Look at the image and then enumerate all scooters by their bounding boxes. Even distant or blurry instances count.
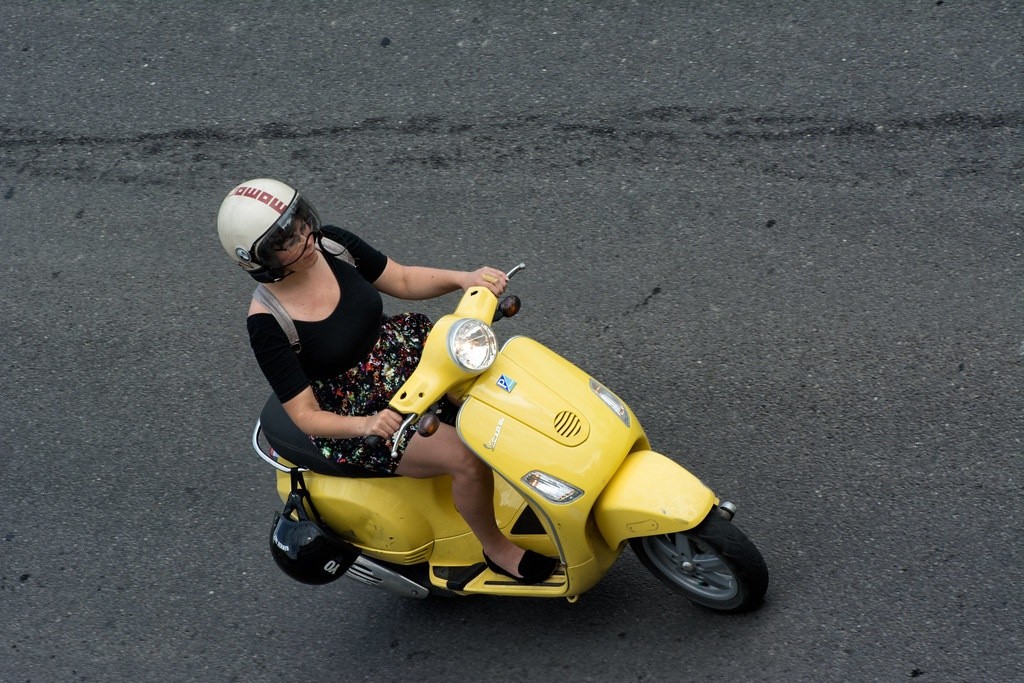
[251,261,771,620]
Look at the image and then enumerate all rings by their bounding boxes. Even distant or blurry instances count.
[482,274,499,284]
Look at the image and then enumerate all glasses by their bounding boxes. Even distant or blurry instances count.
[273,216,313,253]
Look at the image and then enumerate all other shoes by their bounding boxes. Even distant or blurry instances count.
[481,542,560,583]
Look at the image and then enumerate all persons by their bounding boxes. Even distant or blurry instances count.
[215,178,561,585]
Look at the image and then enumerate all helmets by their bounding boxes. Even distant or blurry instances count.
[270,510,362,587]
[217,177,321,285]
